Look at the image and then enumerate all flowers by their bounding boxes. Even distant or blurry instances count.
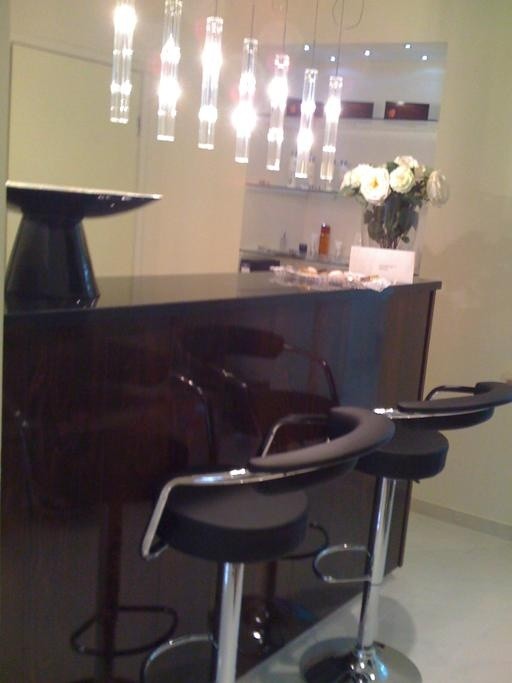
[338,155,449,249]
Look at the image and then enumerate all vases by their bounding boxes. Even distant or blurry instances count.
[350,249,417,284]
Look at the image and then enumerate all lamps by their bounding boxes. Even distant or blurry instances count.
[156,0,183,142]
[296,1,318,177]
[267,1,291,169]
[235,0,260,162]
[198,1,222,149]
[110,0,136,125]
[318,1,344,179]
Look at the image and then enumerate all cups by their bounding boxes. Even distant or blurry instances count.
[298,243,308,256]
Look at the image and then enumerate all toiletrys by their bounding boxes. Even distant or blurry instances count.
[309,231,319,258]
[299,241,308,257]
[278,227,289,256]
[332,238,343,263]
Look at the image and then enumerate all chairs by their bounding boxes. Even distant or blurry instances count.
[300,382,511,683]
[140,405,395,683]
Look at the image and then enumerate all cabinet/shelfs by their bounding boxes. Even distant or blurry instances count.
[240,111,439,271]
